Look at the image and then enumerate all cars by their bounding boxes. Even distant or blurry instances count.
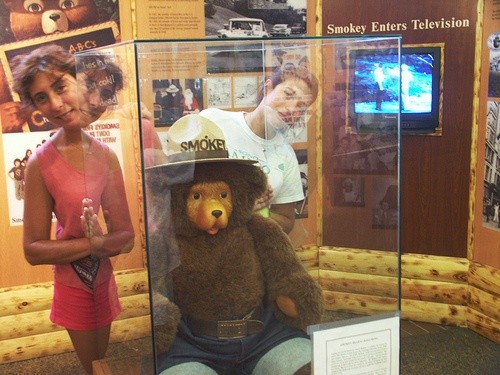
[291,23,305,34]
[270,24,291,37]
[216,17,268,38]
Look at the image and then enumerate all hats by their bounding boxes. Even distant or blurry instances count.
[144,114,258,169]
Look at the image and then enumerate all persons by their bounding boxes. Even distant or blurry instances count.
[401,64,413,110]
[342,183,359,201]
[375,63,384,110]
[14,44,90,371]
[375,201,397,224]
[74,53,136,362]
[158,84,199,121]
[8,140,46,200]
[333,117,397,170]
[485,202,499,222]
[120,65,318,233]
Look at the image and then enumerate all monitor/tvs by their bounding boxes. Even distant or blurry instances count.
[348,47,442,133]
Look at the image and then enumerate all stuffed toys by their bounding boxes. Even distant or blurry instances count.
[108,159,324,375]
